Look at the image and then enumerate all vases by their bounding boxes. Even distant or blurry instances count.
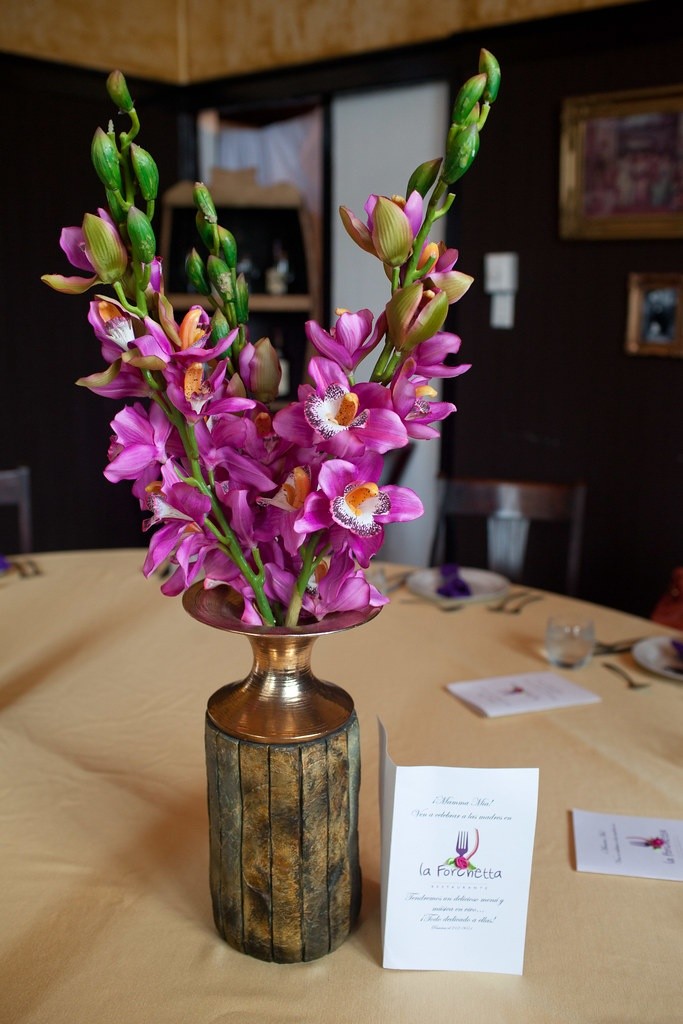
[180,577,385,966]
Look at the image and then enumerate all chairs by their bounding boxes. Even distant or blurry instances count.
[0,466,31,553]
[436,476,587,573]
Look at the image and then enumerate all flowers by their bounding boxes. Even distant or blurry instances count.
[40,47,499,626]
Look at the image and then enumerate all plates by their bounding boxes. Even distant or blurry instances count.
[407,565,512,606]
[632,635,682,680]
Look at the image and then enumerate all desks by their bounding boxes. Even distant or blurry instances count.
[0,551,683,1024]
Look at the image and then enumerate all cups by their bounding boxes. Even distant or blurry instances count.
[546,617,595,670]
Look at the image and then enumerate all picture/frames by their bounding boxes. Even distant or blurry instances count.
[625,272,683,356]
[558,85,683,239]
